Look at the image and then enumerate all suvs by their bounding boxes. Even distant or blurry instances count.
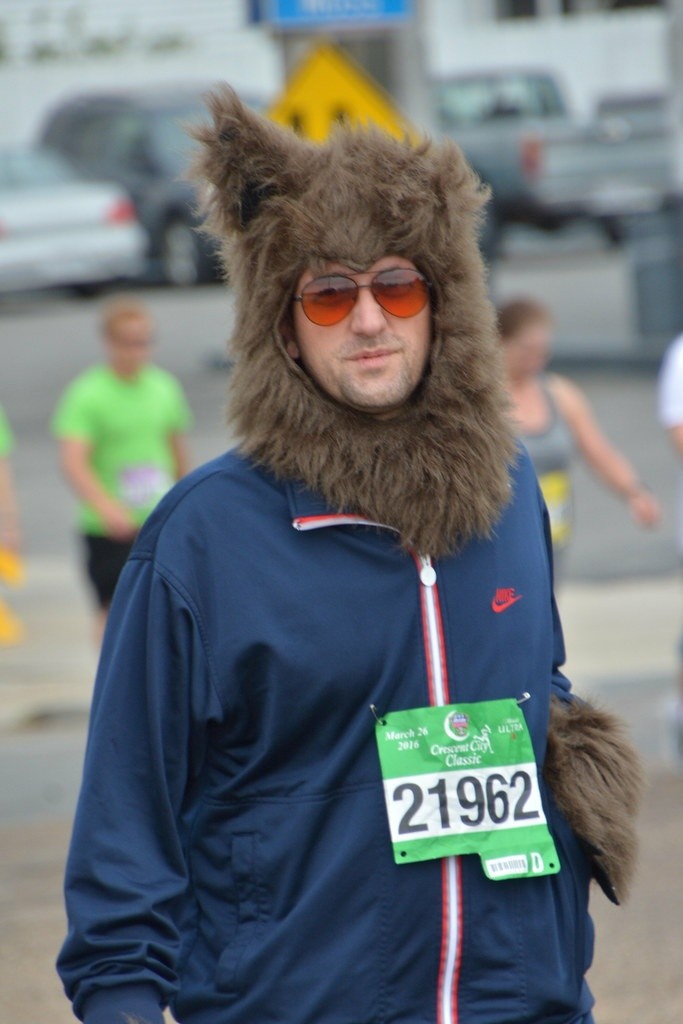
[440,70,683,251]
[2,47,277,299]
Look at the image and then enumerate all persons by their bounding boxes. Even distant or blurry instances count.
[0,408,23,551]
[55,77,660,1024]
[661,333,682,458]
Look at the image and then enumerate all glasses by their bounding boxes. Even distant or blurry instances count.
[292,268,435,327]
